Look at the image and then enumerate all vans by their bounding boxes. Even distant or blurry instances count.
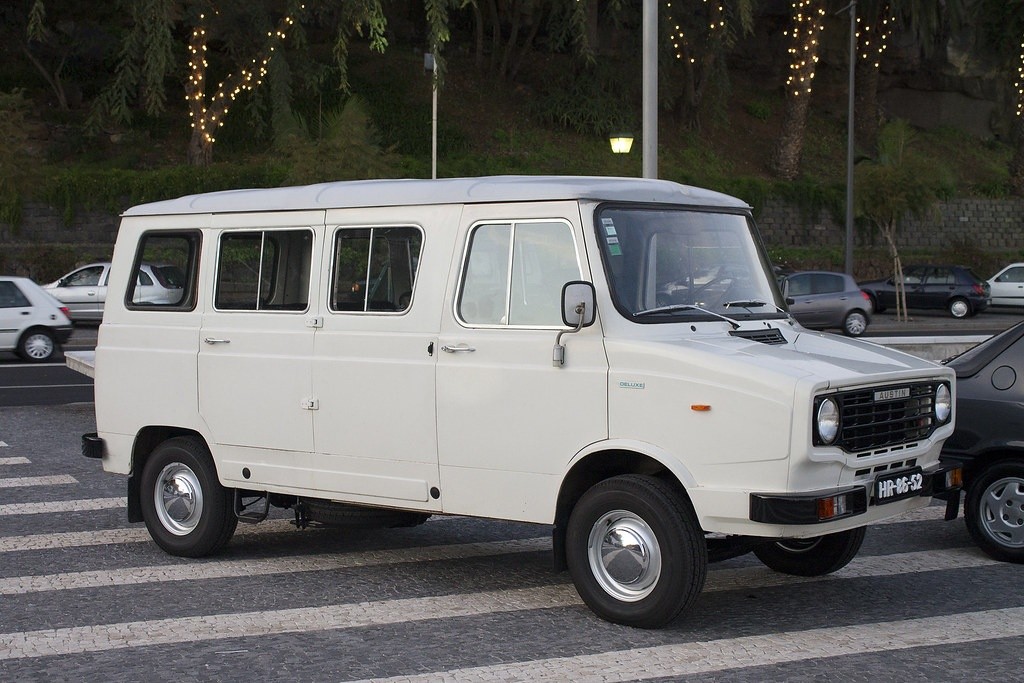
[80,173,959,625]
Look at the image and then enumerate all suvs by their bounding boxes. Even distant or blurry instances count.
[854,264,993,320]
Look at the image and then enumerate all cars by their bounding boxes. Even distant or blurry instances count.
[984,262,1024,308]
[34,261,191,322]
[778,270,874,336]
[0,275,77,361]
[928,316,1024,563]
